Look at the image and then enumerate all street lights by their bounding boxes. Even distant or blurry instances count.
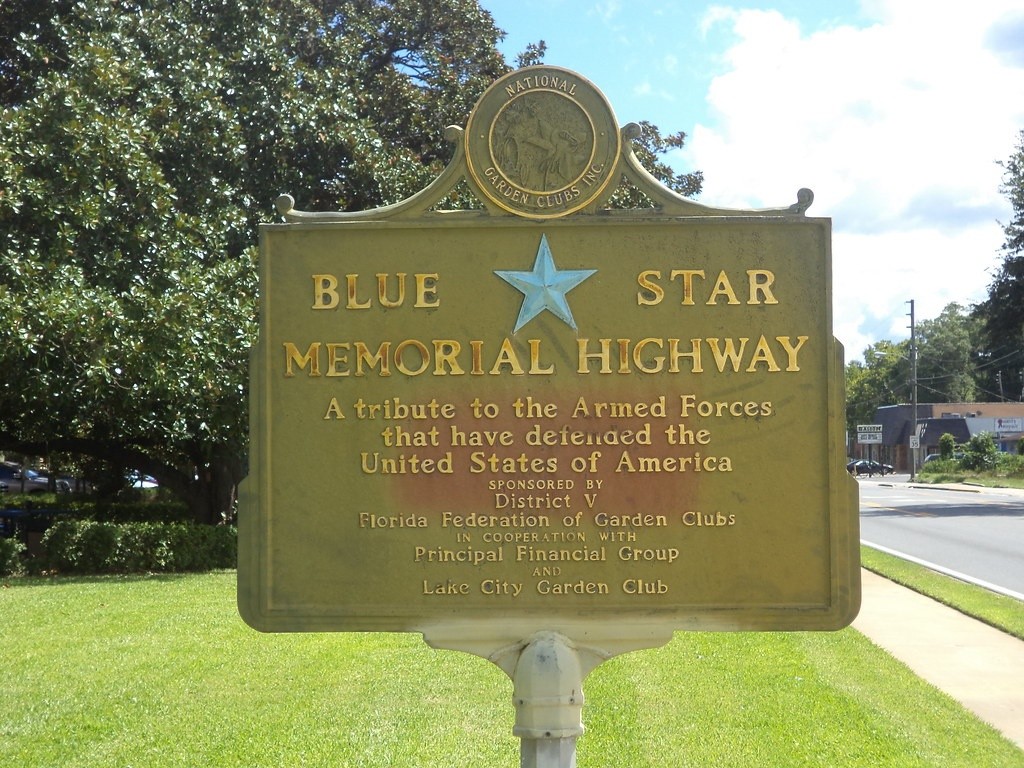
[873,351,919,483]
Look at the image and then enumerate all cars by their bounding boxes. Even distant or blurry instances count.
[854,460,893,475]
[0,461,71,497]
[846,460,860,474]
[925,454,942,464]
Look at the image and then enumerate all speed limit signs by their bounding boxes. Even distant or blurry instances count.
[909,435,919,448]
